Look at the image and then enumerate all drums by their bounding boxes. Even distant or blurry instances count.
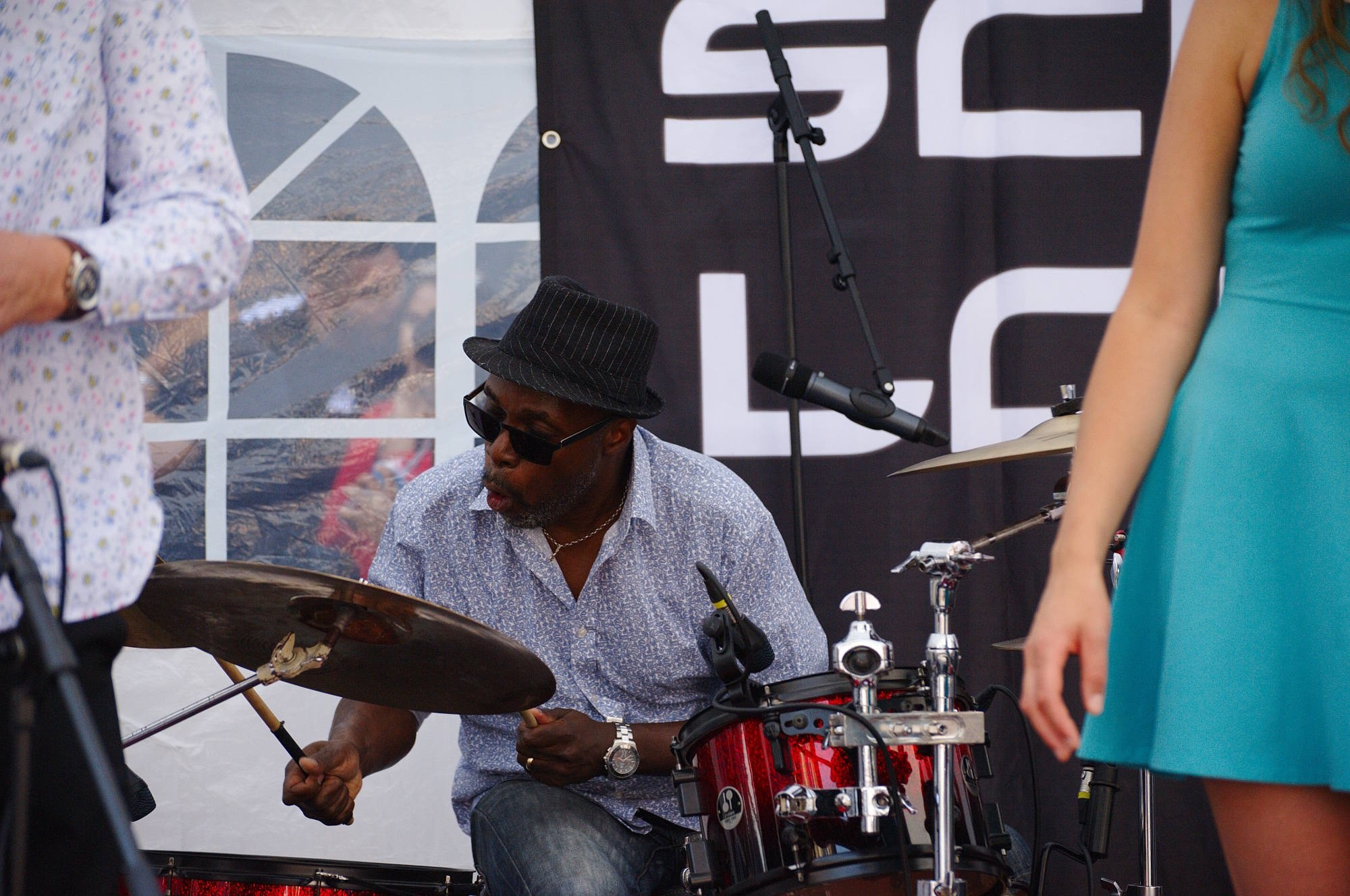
[677,665,1015,896]
[153,854,485,895]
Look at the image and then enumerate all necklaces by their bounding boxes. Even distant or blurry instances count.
[543,480,627,566]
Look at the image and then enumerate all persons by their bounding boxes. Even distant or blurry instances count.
[280,276,831,896]
[321,280,434,579]
[1019,0,1350,896]
[0,0,253,896]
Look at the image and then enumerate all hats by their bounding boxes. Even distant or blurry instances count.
[462,274,665,419]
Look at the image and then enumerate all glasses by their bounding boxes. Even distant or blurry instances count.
[463,377,617,466]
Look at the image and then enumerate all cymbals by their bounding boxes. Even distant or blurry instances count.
[884,412,1081,482]
[138,557,560,718]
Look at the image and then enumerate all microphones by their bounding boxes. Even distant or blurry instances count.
[970,684,996,712]
[0,438,50,475]
[1078,759,1096,828]
[695,561,775,673]
[752,351,950,446]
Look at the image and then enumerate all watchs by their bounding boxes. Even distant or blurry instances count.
[57,235,103,322]
[605,721,639,779]
